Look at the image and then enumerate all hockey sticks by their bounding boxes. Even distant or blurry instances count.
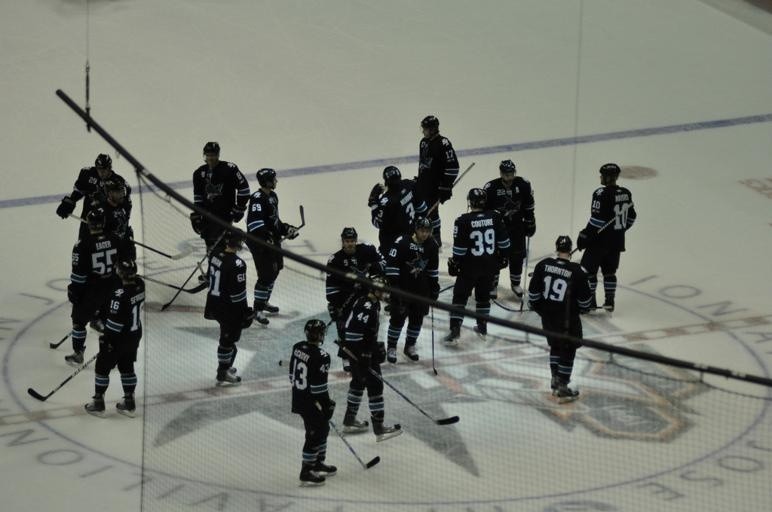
[313,400,380,471]
[431,303,440,376]
[139,274,210,294]
[49,330,72,349]
[341,346,460,426]
[278,291,355,366]
[27,356,98,401]
[273,205,305,243]
[69,213,193,261]
[519,236,531,312]
[160,218,236,311]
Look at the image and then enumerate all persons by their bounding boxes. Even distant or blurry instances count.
[204,229,255,387]
[483,159,536,299]
[412,116,460,247]
[288,320,337,486]
[190,142,250,283]
[326,226,388,361]
[529,236,595,402]
[342,274,404,441]
[65,209,128,368]
[580,164,636,311]
[90,184,137,336]
[442,188,511,345]
[85,257,144,418]
[248,168,298,328]
[56,154,132,240]
[384,214,440,367]
[367,167,429,256]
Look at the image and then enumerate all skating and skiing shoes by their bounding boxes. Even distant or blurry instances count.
[65,349,86,368]
[603,298,615,318]
[386,342,398,366]
[217,363,243,386]
[342,357,354,377]
[299,466,328,486]
[84,397,107,419]
[374,417,403,442]
[114,397,137,418]
[511,284,526,298]
[473,325,488,340]
[549,376,579,404]
[342,414,370,435]
[490,285,498,300]
[402,343,420,363]
[255,307,269,328]
[318,463,336,477]
[263,303,281,316]
[443,325,461,346]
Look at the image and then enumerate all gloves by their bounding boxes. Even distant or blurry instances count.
[328,302,341,320]
[374,341,386,364]
[240,306,254,330]
[57,196,77,219]
[523,218,536,235]
[91,313,106,333]
[430,281,441,300]
[281,224,299,238]
[190,211,207,236]
[318,398,335,420]
[577,228,598,251]
[230,207,247,222]
[68,282,87,304]
[448,258,460,275]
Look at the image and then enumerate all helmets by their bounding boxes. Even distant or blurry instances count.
[556,235,572,250]
[303,319,326,341]
[87,211,106,232]
[203,143,220,158]
[115,257,138,277]
[383,165,402,183]
[422,116,441,128]
[499,159,517,172]
[340,227,358,240]
[257,168,276,185]
[414,216,434,231]
[600,163,622,176]
[470,188,488,204]
[104,176,126,193]
[95,155,114,167]
